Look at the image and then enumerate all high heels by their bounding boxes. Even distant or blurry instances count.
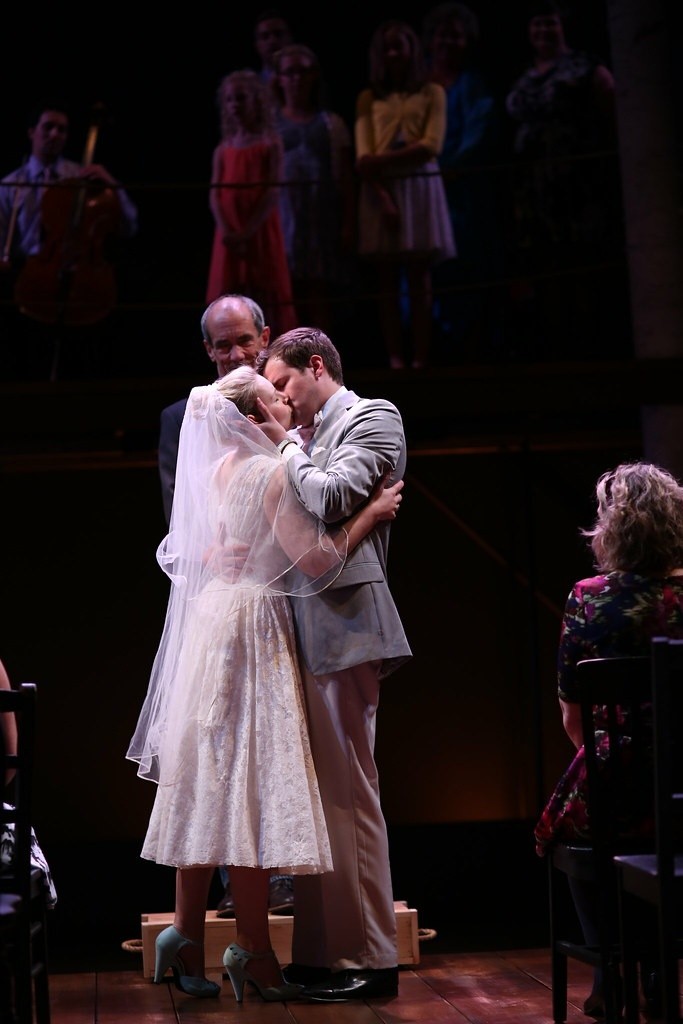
[153,926,221,998]
[223,942,305,1003]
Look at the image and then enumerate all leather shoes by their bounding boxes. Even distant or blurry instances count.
[303,969,399,1002]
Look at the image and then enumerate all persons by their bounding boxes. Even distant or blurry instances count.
[535,465,683,1024]
[1,102,139,383]
[0,658,56,1024]
[126,295,406,1004]
[210,1,614,377]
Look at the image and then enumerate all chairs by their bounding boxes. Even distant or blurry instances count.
[0,682,52,1024]
[547,637,683,1024]
